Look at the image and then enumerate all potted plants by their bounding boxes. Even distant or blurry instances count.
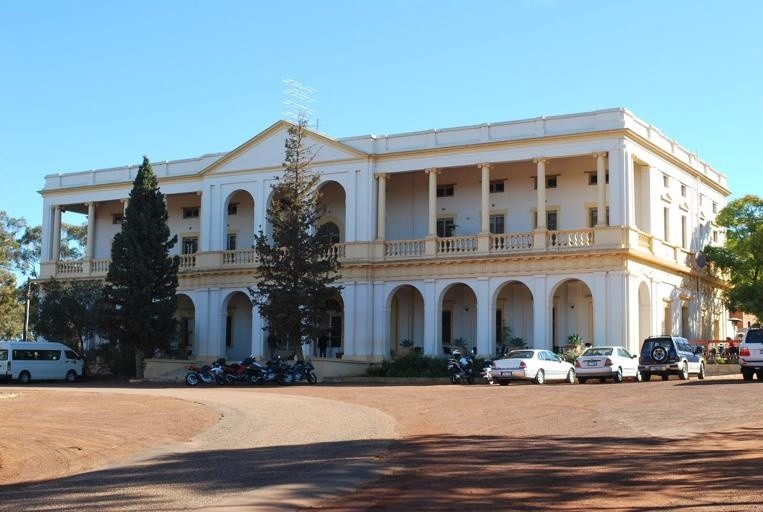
[453,337,469,356]
[564,333,584,353]
[506,337,526,352]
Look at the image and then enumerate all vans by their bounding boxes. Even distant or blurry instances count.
[0,341,86,384]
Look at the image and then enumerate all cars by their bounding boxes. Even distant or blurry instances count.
[479,335,642,386]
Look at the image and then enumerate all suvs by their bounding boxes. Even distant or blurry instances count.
[638,334,706,380]
[738,327,763,382]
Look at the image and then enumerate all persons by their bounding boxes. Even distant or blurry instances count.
[317,334,329,357]
[266,334,282,360]
[722,337,736,356]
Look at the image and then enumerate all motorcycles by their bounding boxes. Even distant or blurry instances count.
[447,350,479,384]
[185,352,317,386]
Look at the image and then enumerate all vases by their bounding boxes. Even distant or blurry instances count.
[399,340,413,356]
